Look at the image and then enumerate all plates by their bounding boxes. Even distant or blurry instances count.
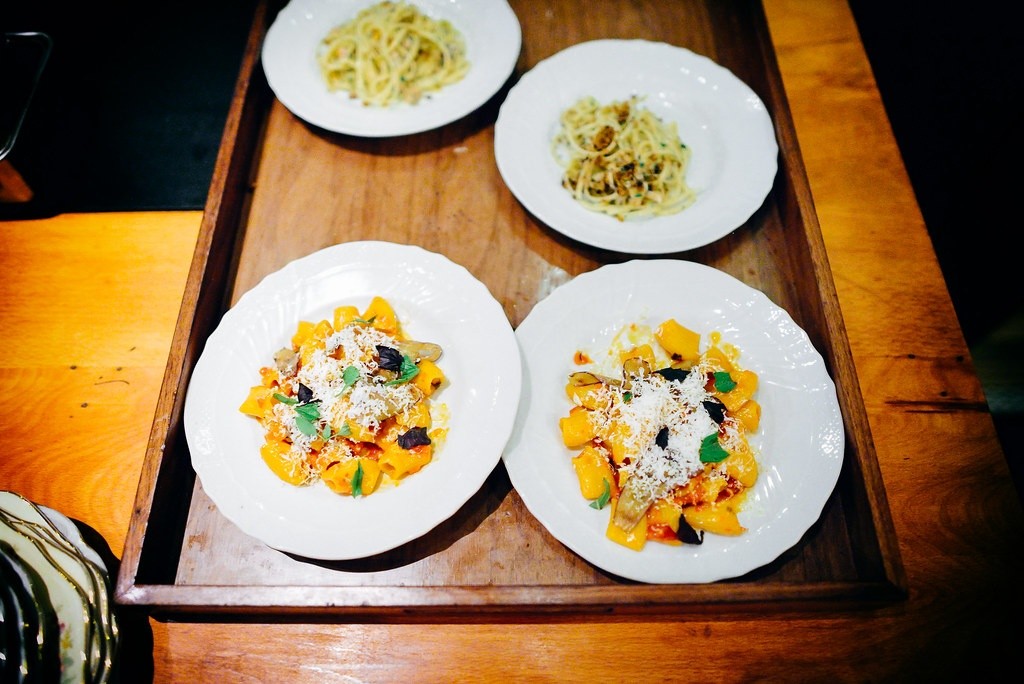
[493,39,778,254]
[500,258,845,583]
[182,241,524,562]
[0,488,119,684]
[260,0,522,138]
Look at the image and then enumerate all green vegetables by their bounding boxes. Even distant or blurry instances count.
[275,313,421,500]
[588,371,737,510]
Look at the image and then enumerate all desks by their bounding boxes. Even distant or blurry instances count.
[0,0,1024,684]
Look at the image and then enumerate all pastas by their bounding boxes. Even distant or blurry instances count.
[318,0,470,107]
[553,95,696,222]
